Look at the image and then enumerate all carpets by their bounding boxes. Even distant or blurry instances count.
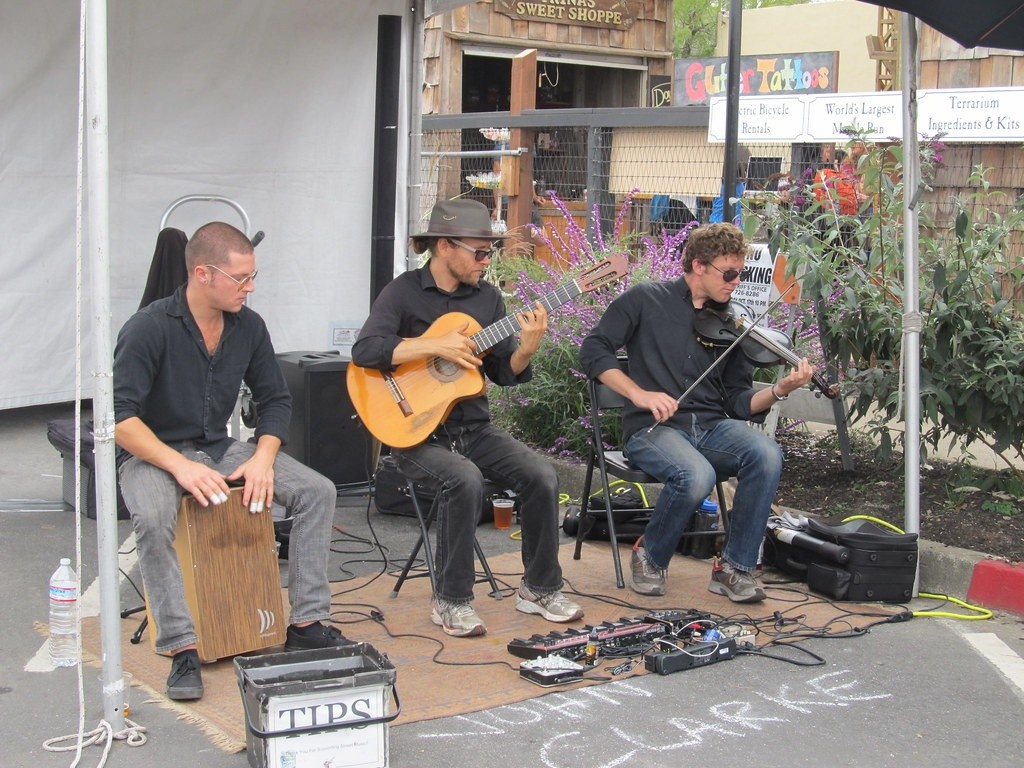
[28,531,920,757]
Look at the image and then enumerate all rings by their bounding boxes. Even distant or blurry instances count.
[652,408,657,411]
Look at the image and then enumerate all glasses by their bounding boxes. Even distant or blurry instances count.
[704,258,751,282]
[202,263,259,292]
[446,236,495,261]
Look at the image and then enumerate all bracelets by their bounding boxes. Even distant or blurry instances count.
[772,384,790,401]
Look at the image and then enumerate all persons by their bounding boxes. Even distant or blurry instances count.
[113,221,358,699]
[710,163,745,229]
[351,199,585,637]
[492,142,545,229]
[580,222,817,601]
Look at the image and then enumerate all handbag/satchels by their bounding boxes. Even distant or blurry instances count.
[801,515,917,603]
[47,418,94,476]
[585,479,697,549]
[373,451,522,526]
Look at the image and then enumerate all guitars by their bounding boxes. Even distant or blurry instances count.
[345,251,631,451]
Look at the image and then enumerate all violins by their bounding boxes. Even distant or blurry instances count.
[693,299,843,401]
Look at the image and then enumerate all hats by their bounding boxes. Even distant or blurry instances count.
[409,198,511,238]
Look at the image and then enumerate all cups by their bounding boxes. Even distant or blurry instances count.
[492,499,515,530]
[97,672,133,716]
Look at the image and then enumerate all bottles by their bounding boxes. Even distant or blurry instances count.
[690,498,720,559]
[756,541,762,570]
[48,558,78,667]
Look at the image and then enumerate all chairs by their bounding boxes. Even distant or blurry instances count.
[574,351,732,591]
[391,454,505,601]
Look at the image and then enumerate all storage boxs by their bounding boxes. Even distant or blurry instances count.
[225,640,398,768]
[140,485,289,662]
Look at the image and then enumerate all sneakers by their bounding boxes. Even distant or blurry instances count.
[285,622,360,651]
[166,645,204,700]
[515,576,585,622]
[430,591,487,637]
[630,531,669,596]
[708,556,769,604]
